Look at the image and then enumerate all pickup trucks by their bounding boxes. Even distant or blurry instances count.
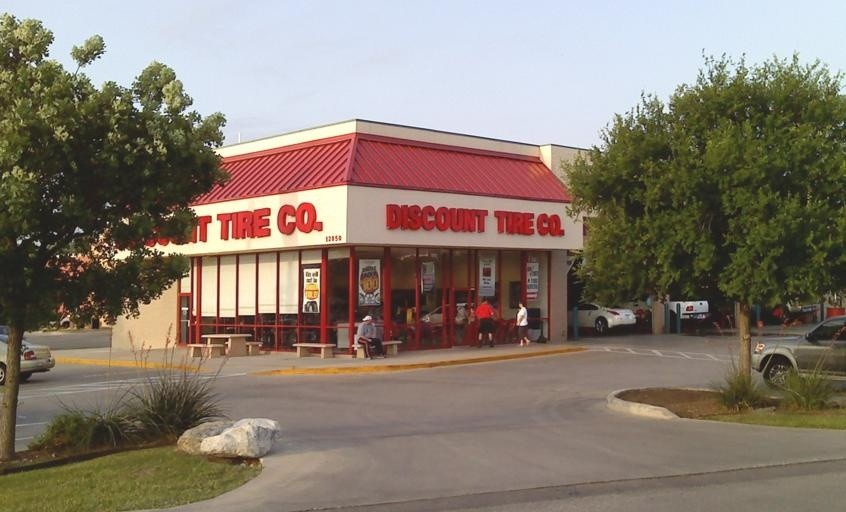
[647,293,709,320]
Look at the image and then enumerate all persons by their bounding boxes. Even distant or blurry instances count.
[514,300,531,347]
[455,307,468,336]
[356,315,384,359]
[475,297,495,349]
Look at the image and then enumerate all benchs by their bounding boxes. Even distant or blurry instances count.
[352,340,402,358]
[291,342,337,358]
[188,341,263,358]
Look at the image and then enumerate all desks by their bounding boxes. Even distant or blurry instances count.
[201,333,252,357]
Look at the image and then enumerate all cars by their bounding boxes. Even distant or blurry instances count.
[0,325,56,385]
[60,315,80,328]
[751,315,846,390]
[420,303,475,328]
[568,301,645,336]
[267,318,316,349]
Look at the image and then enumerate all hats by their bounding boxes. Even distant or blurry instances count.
[362,315,373,321]
